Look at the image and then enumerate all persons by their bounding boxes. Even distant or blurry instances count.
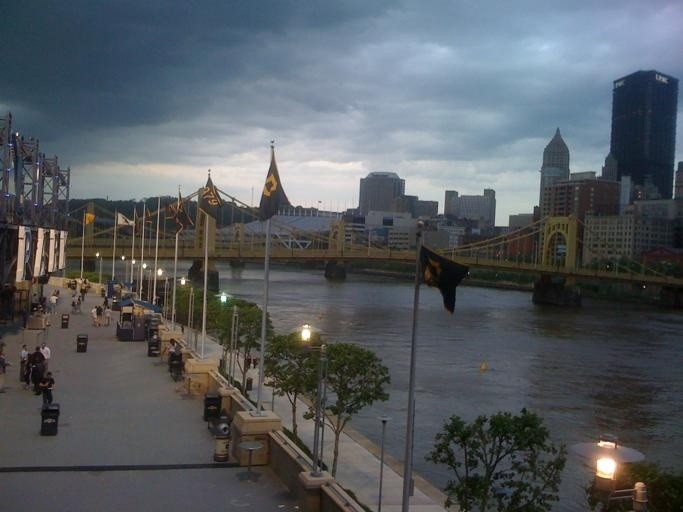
[18,344,27,381]
[28,346,45,392]
[166,338,177,376]
[38,371,55,404]
[29,278,111,327]
[38,341,51,371]
[0,343,12,394]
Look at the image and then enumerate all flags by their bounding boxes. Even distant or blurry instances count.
[134,211,142,235]
[257,149,292,221]
[175,192,193,234]
[197,177,223,221]
[117,211,134,227]
[417,244,470,317]
[85,213,98,225]
[165,202,177,219]
[144,207,151,225]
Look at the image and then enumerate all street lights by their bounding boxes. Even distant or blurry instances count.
[214,290,239,391]
[297,319,330,478]
[92,249,196,352]
[569,430,651,512]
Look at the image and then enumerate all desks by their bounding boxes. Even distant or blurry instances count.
[237,441,264,482]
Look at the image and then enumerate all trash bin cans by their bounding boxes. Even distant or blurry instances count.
[214,422,230,462]
[204,394,220,422]
[77,334,88,352]
[61,313,69,329]
[41,403,60,436]
